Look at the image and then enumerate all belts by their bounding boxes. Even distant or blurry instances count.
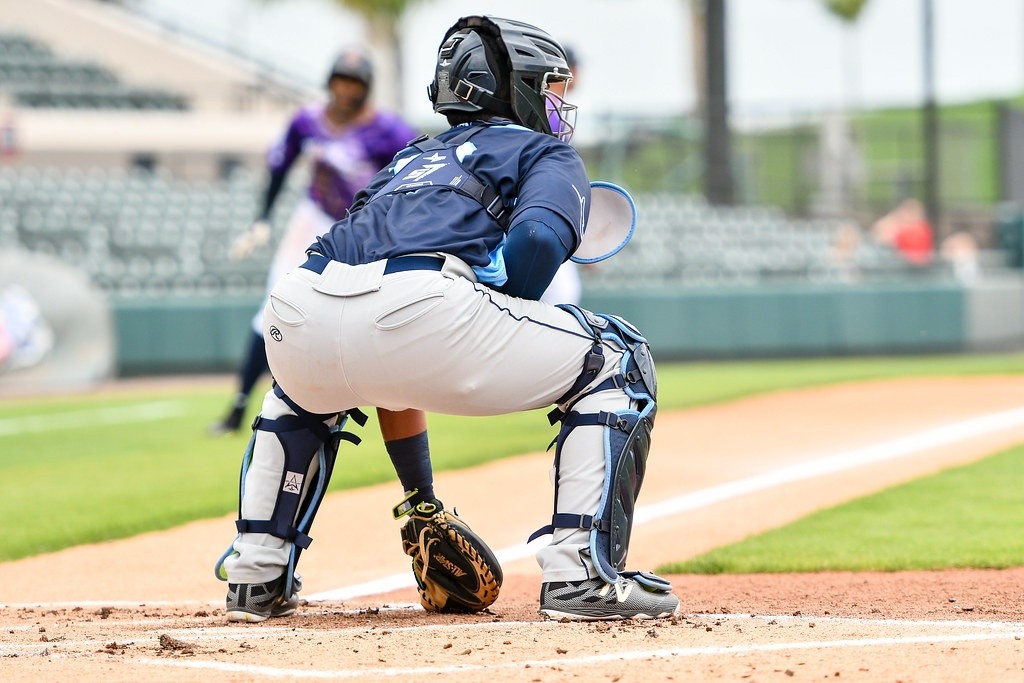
[298,254,445,275]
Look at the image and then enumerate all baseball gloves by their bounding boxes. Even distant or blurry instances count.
[394,498,504,613]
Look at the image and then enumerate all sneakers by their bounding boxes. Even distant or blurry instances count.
[536,576,680,620]
[225,577,298,622]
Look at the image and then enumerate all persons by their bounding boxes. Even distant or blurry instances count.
[214,49,421,437]
[217,12,681,619]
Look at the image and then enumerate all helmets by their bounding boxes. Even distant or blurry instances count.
[332,51,374,86]
[426,15,578,143]
